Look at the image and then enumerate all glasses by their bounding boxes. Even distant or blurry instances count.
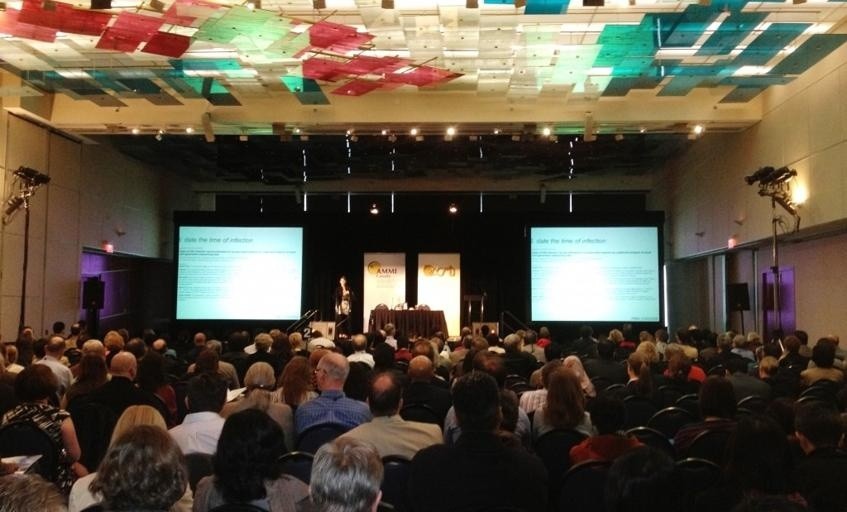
[314,369,327,374]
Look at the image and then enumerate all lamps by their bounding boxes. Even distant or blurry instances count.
[347,125,555,213]
[132,125,194,141]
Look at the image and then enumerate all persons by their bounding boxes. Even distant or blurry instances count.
[335,276,352,339]
[0,322,847,512]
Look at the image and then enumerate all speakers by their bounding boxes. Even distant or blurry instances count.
[727,282,751,311]
[82,276,105,309]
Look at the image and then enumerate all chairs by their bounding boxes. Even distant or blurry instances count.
[0,343,847,512]
